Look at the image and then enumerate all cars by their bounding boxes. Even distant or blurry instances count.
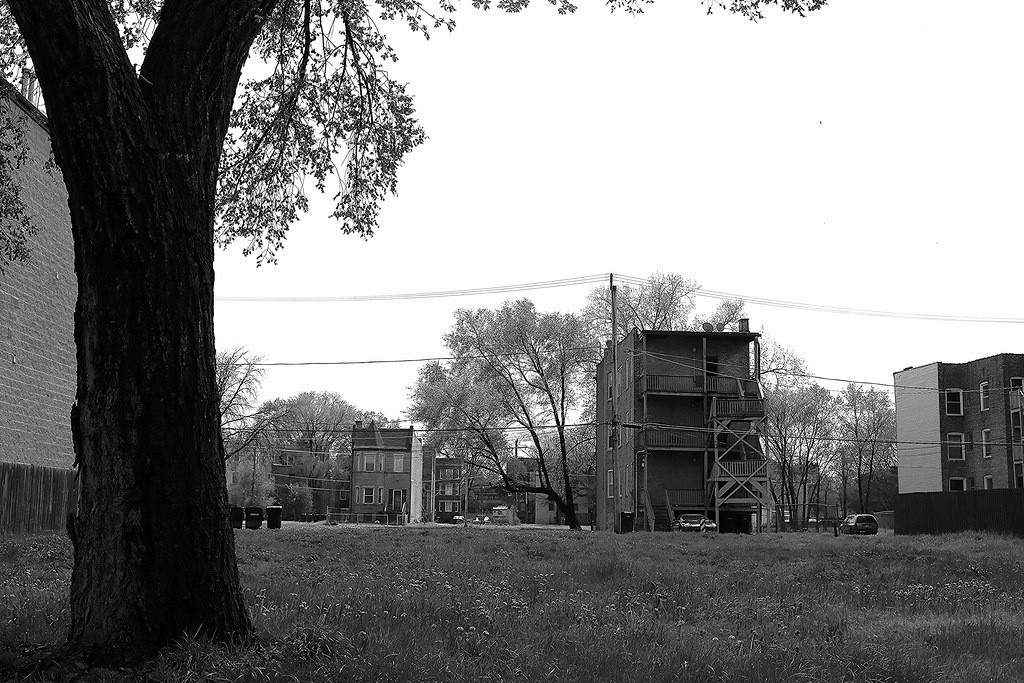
[673,515,717,533]
[453,515,464,525]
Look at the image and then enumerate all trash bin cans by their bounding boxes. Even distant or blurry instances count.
[245,505,262,530]
[621,511,634,533]
[267,506,282,529]
[230,504,244,530]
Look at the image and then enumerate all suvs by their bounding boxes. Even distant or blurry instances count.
[842,514,879,536]
